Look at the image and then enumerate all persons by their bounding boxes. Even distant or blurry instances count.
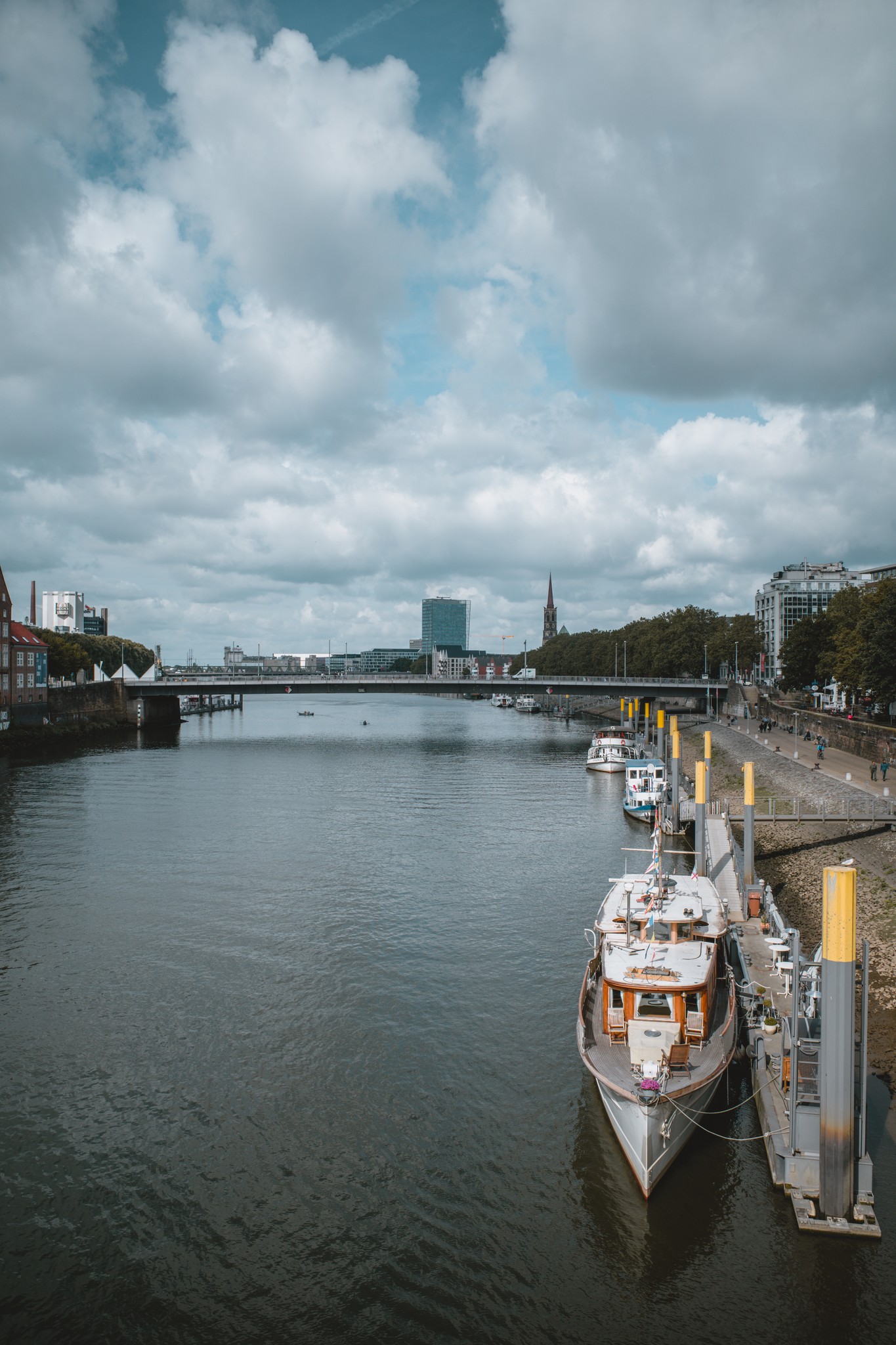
[304,711,310,713]
[869,761,878,782]
[764,717,768,723]
[865,703,874,711]
[321,672,324,680]
[726,714,737,726]
[771,720,776,726]
[712,709,715,717]
[880,759,890,781]
[725,677,728,685]
[754,701,758,711]
[743,678,746,686]
[802,729,812,741]
[756,679,758,687]
[333,672,344,680]
[758,721,772,733]
[788,725,794,733]
[817,743,825,755]
[364,721,367,724]
[813,734,826,747]
[847,713,854,721]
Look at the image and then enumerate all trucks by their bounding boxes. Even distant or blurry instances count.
[512,668,536,680]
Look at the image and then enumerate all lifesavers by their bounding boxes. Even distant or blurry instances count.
[560,708,562,711]
[197,695,198,696]
[621,739,625,745]
[598,739,601,746]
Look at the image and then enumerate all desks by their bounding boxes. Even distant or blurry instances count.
[765,938,785,970]
[775,962,793,998]
[768,945,790,978]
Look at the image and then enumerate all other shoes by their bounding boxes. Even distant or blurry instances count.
[874,780,876,781]
[883,779,885,781]
[871,779,873,781]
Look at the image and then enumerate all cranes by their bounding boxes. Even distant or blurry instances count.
[467,635,514,654]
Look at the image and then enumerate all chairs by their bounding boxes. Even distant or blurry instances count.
[684,1011,705,1052]
[661,1044,691,1080]
[607,1008,628,1047]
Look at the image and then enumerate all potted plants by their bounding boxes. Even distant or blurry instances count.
[725,908,731,926]
[637,783,642,792]
[758,908,769,935]
[656,782,660,792]
[755,986,778,1035]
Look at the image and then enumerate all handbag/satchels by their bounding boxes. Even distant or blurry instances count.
[758,728,760,732]
[766,726,769,729]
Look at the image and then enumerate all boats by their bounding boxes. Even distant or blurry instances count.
[586,726,743,1201]
[179,694,226,710]
[462,693,577,718]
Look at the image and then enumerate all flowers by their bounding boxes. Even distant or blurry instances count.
[640,1079,659,1091]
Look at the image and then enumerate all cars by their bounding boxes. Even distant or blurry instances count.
[247,671,326,672]
[372,671,411,673]
[175,671,215,673]
[738,679,752,687]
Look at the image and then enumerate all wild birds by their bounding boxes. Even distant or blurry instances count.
[840,858,855,868]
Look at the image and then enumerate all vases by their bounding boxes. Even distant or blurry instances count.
[642,1089,657,1096]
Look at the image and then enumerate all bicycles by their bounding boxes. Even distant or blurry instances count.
[817,750,824,760]
[332,676,340,681]
[622,680,728,684]
[711,715,721,722]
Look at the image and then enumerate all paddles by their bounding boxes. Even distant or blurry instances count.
[360,722,370,724]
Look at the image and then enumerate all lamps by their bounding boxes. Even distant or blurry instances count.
[681,991,687,1003]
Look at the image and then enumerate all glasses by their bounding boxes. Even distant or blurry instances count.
[873,762,875,763]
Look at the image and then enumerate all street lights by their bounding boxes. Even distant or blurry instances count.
[328,639,347,679]
[426,638,437,680]
[710,694,713,718]
[792,711,800,752]
[233,641,236,681]
[735,642,739,681]
[746,700,750,729]
[704,645,708,674]
[122,642,125,679]
[615,644,618,682]
[624,641,627,679]
[257,643,260,680]
